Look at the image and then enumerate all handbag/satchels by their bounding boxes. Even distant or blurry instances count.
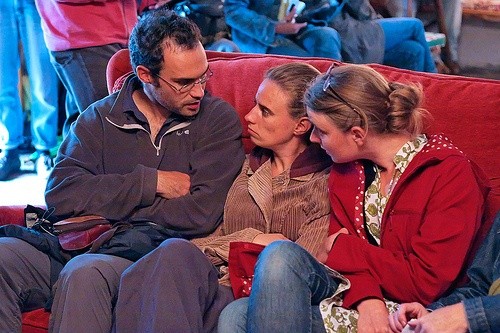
[52,215,132,254]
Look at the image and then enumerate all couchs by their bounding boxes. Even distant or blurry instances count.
[0,48,500,333]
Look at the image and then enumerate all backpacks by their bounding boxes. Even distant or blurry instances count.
[172,2,231,42]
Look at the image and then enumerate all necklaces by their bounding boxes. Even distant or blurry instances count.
[387,164,398,199]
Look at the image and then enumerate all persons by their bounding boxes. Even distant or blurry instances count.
[218,65,495,333]
[0,9,248,333]
[111,64,338,333]
[386,209,500,333]
[0,0,463,181]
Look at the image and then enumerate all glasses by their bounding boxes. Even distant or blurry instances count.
[153,67,214,94]
[322,62,365,127]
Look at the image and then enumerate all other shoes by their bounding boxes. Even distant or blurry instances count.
[36,154,54,181]
[433,59,460,73]
[0,150,21,181]
